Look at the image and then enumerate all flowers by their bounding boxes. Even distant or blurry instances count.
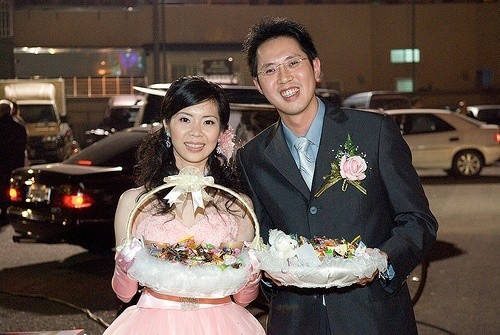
[314,132,370,198]
[216,125,238,160]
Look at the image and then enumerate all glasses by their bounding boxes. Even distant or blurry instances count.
[257,57,308,78]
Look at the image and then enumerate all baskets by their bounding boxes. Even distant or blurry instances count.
[126,182,259,299]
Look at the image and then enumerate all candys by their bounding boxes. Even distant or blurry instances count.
[146,235,250,269]
[285,232,367,259]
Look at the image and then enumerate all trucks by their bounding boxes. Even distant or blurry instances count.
[0,78,68,166]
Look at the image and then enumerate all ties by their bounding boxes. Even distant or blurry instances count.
[294,138,315,192]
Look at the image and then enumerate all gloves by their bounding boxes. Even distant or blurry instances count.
[111,246,143,298]
[233,251,262,304]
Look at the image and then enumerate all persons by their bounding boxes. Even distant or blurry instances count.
[0,98,29,225]
[236,15,439,335]
[101,75,266,335]
[457,101,474,118]
[410,97,423,108]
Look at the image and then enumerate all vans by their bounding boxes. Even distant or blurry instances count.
[135,77,342,130]
[340,90,414,110]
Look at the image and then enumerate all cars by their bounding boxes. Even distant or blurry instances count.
[363,109,500,180]
[84,95,145,146]
[8,121,178,253]
[466,104,500,125]
